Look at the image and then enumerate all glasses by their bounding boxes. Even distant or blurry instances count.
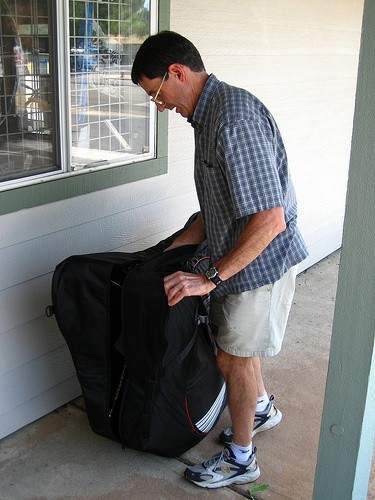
[151,72,167,105]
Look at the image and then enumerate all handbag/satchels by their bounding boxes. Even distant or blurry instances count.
[45,211,229,457]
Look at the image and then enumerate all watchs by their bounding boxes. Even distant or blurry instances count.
[206,266,221,285]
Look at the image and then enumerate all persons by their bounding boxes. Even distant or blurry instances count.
[129,32,309,488]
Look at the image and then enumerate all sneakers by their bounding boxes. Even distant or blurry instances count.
[184,447,260,488]
[218,395,282,444]
